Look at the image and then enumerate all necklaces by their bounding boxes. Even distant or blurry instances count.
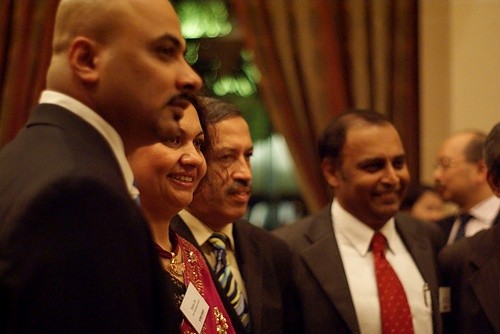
[145,223,188,276]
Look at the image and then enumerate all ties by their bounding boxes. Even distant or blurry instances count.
[206,231,252,334]
[368,231,415,334]
[453,214,472,242]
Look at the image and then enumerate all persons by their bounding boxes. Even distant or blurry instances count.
[0,1,204,334]
[112,92,249,333]
[436,122,500,334]
[268,109,453,334]
[169,98,352,334]
[427,129,500,263]
[397,185,447,222]
